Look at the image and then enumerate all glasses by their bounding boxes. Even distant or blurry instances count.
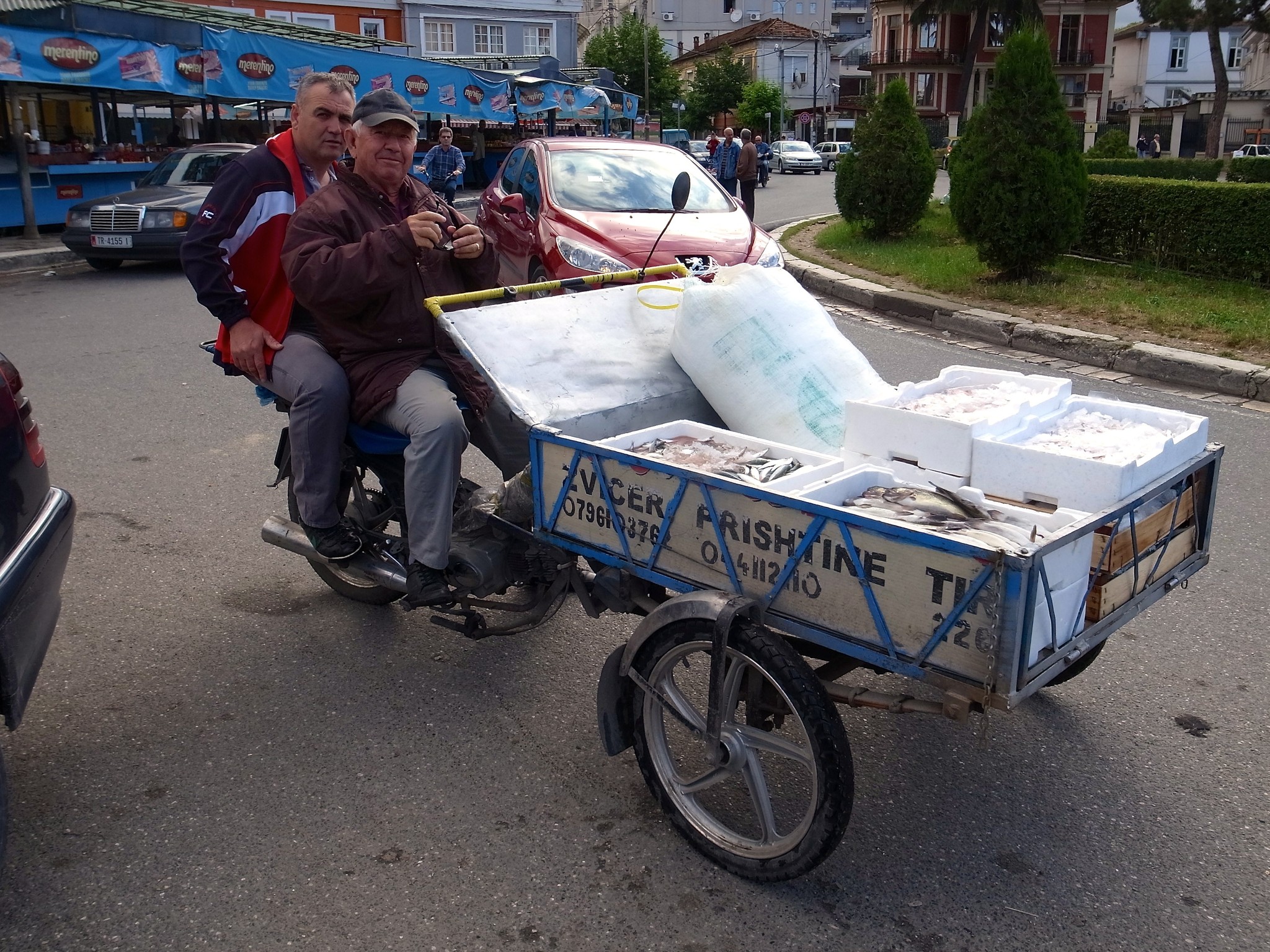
[756,140,761,141]
[441,136,452,138]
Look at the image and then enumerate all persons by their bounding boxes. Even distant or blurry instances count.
[478,119,492,142]
[706,133,720,161]
[197,121,228,144]
[57,125,81,145]
[568,123,585,137]
[179,71,368,567]
[712,128,740,197]
[735,128,758,222]
[234,125,256,144]
[415,127,466,210]
[467,123,491,190]
[754,135,771,182]
[281,87,600,609]
[168,125,184,147]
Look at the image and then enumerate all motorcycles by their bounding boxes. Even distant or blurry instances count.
[203,172,1227,891]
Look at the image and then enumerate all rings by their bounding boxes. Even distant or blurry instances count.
[474,243,480,252]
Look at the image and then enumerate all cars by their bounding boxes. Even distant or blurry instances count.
[932,137,967,170]
[663,129,768,173]
[0,348,78,739]
[62,143,266,274]
[813,142,852,171]
[765,140,823,175]
[1229,144,1270,159]
[476,136,785,303]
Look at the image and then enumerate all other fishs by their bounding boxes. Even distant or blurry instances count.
[628,435,804,485]
[838,480,1054,551]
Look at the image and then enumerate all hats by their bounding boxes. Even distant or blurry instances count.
[351,88,420,132]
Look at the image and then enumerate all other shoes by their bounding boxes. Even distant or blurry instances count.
[471,185,482,190]
[483,183,491,189]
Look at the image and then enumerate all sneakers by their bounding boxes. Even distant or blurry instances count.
[299,514,363,560]
[406,562,452,606]
